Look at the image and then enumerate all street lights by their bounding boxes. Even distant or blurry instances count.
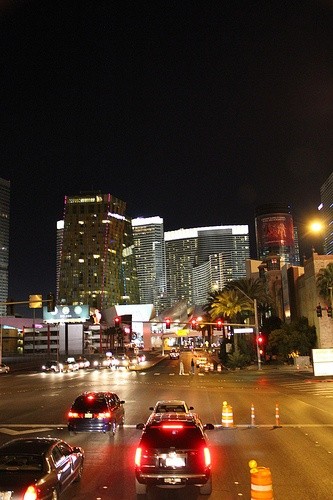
[214,283,261,371]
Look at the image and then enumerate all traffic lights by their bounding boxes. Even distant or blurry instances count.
[317,306,322,317]
[115,317,120,328]
[166,319,170,329]
[326,305,332,317]
[217,320,222,331]
[259,349,263,355]
[258,337,262,347]
[192,319,196,330]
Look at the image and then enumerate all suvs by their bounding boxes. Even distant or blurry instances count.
[68,391,125,438]
[134,412,215,495]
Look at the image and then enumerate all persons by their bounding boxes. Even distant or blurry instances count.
[189,358,195,374]
[179,360,185,375]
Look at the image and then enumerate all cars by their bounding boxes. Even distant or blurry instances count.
[170,348,180,359]
[149,400,194,412]
[0,437,85,500]
[0,363,10,374]
[41,352,146,373]
